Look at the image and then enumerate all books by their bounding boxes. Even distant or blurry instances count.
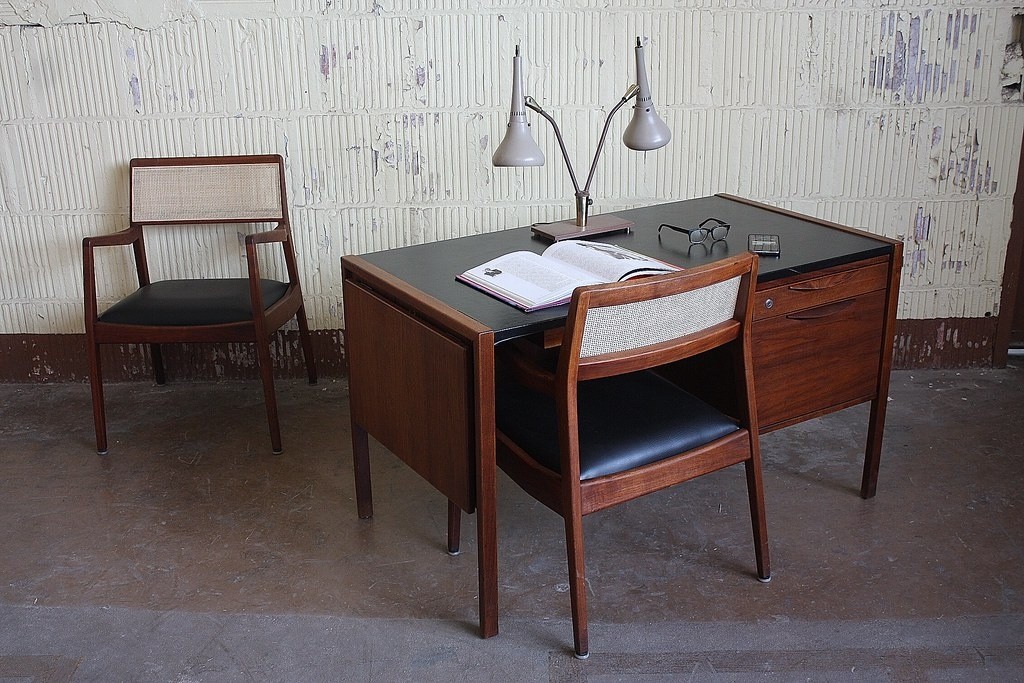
[456,240,685,314]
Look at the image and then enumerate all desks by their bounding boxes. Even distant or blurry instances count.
[340,193,904,640]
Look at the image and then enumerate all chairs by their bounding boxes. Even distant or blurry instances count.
[82,153,318,456]
[445,250,774,659]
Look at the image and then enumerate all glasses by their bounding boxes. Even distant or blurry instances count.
[658,216,731,244]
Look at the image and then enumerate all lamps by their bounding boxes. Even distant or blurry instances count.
[490,34,673,241]
